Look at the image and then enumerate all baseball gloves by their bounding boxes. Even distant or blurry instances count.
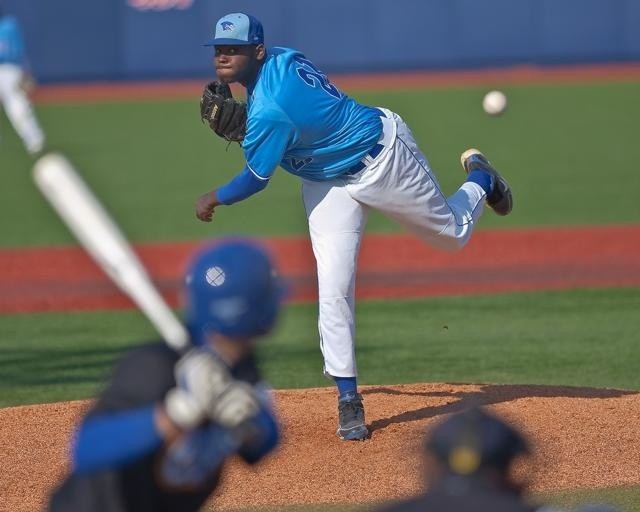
[199,80,247,150]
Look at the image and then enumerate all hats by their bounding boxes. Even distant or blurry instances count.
[202,13,265,48]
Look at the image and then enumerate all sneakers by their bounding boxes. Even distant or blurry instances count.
[460,150,512,216]
[338,393,369,440]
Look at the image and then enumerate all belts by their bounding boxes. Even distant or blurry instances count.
[346,108,389,175]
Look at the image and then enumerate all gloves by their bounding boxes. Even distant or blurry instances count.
[166,356,258,430]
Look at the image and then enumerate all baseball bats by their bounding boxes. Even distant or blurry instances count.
[29,153,256,429]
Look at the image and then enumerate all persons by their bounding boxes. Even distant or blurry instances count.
[378,405,545,511]
[196,12,511,440]
[45,238,294,512]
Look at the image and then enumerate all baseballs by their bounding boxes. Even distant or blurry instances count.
[483,91,507,114]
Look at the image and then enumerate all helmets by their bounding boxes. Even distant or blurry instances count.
[431,416,532,500]
[186,243,283,338]
